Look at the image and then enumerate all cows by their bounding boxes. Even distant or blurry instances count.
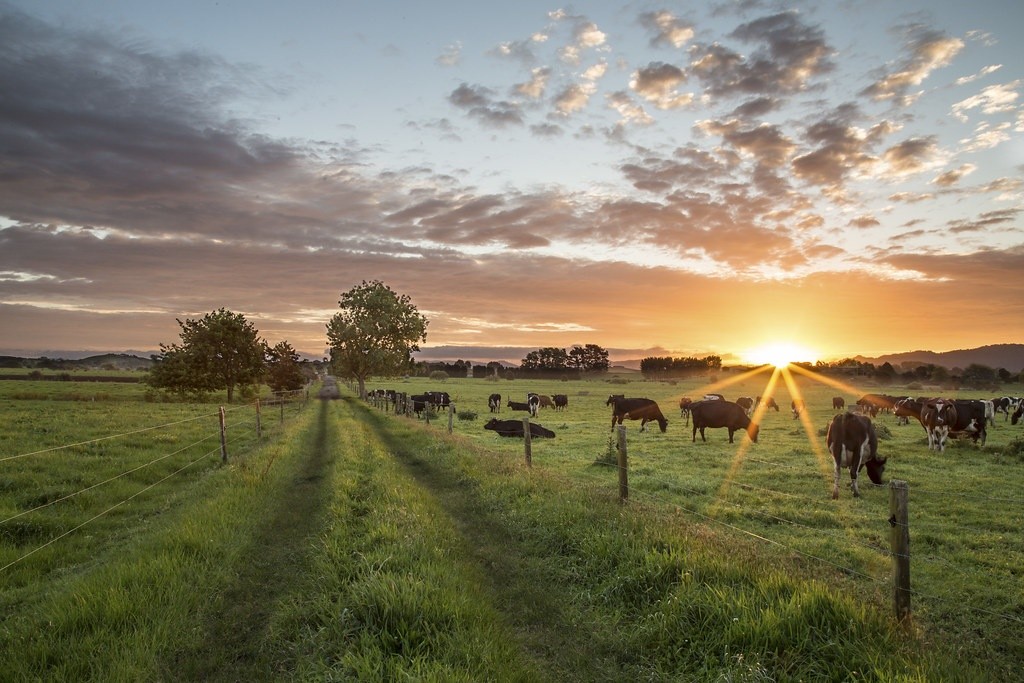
[368,389,1024,502]
